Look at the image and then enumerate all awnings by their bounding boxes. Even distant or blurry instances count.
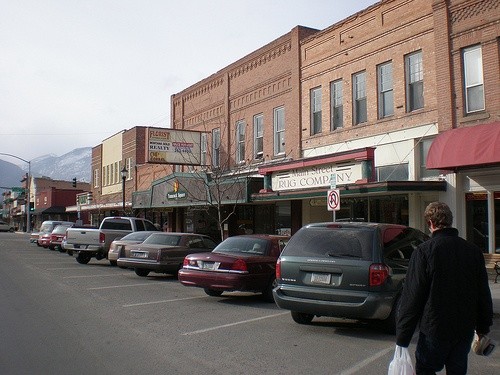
[425,120,500,169]
[36,207,65,215]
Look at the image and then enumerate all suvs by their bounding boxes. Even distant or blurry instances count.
[271,221,432,336]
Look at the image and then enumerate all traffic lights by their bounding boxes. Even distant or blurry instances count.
[30,202,34,211]
[72,177,77,187]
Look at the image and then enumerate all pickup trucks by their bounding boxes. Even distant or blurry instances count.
[64,216,159,264]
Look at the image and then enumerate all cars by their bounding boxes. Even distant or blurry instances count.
[115,232,219,278]
[0,221,16,232]
[177,233,292,303]
[107,230,160,268]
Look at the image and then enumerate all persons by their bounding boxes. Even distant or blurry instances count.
[30,221,34,230]
[396,202,492,375]
[154,222,167,231]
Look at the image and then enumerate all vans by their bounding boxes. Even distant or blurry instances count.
[29,220,71,253]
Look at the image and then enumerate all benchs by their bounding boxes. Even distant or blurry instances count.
[483,254,500,283]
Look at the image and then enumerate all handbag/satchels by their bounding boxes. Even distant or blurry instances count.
[388,345,416,375]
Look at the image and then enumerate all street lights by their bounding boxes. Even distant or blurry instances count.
[120,167,128,217]
